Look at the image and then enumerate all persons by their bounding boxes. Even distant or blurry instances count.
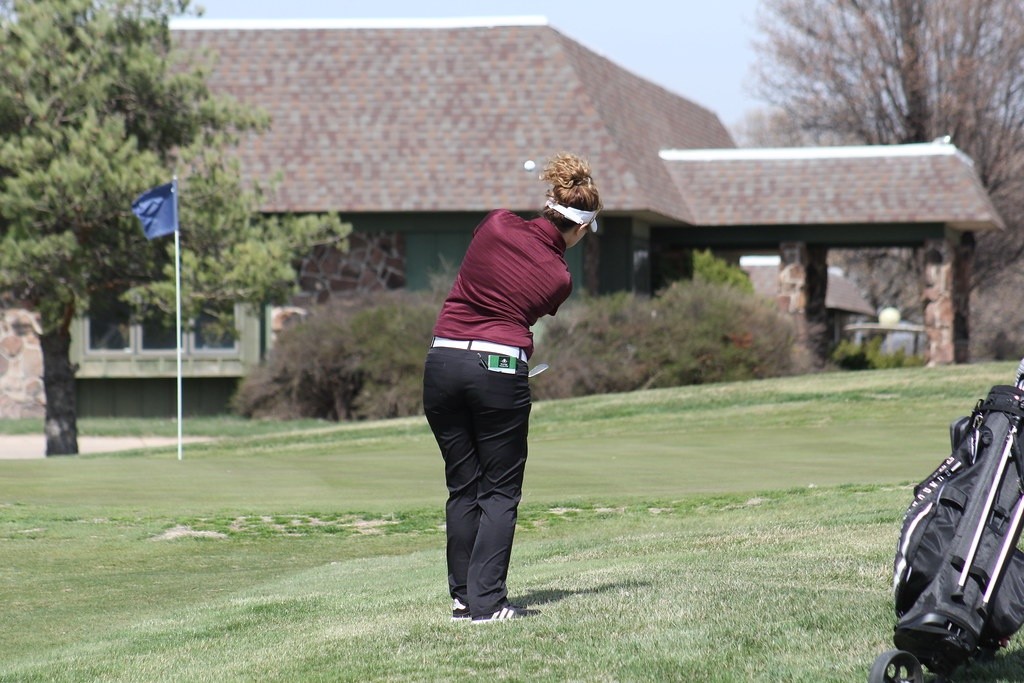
[423,152,605,625]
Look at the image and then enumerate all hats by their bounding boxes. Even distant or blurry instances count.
[546,196,601,232]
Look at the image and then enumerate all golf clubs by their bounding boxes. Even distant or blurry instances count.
[983,496,1024,605]
[951,358,1024,595]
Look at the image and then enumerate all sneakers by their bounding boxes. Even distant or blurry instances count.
[451,600,540,624]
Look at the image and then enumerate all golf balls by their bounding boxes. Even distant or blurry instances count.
[524,160,536,171]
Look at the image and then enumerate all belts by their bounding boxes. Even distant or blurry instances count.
[430,336,528,364]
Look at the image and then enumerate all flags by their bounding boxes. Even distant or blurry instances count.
[132,180,181,241]
